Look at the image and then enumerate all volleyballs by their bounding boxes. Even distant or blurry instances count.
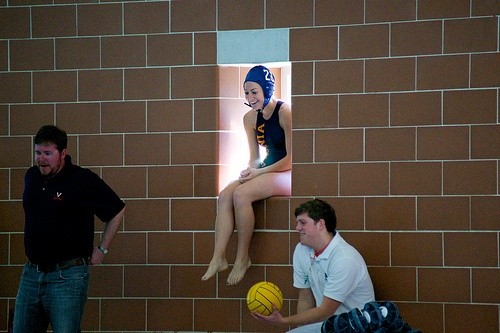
[247,280,284,315]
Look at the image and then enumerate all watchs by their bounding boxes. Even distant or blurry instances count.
[97,245,110,255]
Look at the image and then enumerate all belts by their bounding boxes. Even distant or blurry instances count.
[25,256,89,272]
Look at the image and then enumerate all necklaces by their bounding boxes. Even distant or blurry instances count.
[304,254,319,284]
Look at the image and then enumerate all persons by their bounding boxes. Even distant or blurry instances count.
[200,65,292,285]
[250,199,376,333]
[12,125,126,333]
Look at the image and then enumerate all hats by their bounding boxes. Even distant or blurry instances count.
[243,65,275,109]
